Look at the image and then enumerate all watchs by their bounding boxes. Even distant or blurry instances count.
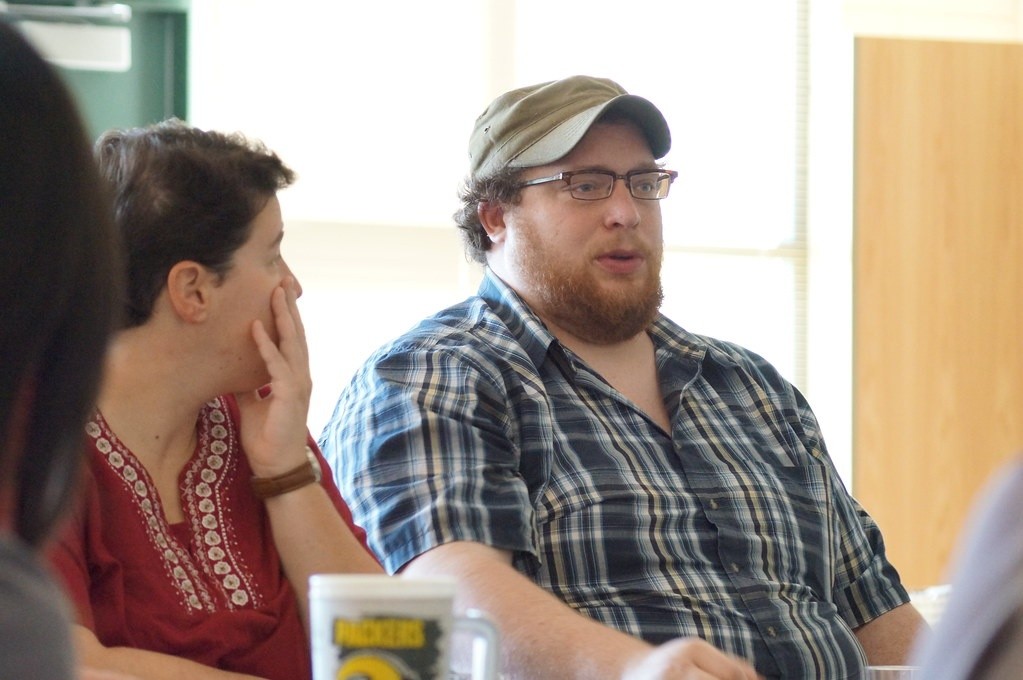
[246,446,321,499]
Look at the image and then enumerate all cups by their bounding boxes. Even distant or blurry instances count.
[305,571,501,680]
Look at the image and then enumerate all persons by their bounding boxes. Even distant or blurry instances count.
[316,71,945,680]
[33,119,392,680]
[0,7,121,679]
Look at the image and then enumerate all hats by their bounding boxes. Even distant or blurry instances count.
[466,75,671,186]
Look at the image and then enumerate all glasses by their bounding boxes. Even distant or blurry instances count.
[515,167,679,201]
